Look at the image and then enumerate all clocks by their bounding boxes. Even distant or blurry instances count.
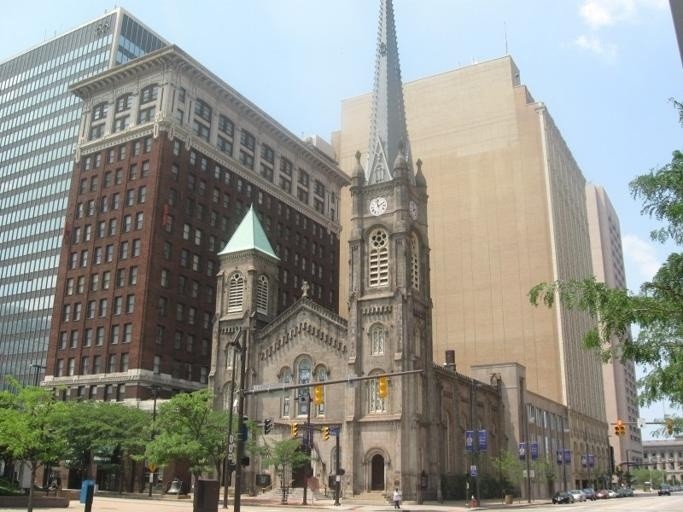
[368,197,386,217]
[408,200,417,220]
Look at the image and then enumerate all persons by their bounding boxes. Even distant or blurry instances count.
[394,488,402,509]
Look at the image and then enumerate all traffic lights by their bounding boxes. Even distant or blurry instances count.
[290,422,299,439]
[380,378,389,395]
[264,418,272,435]
[321,426,330,440]
[667,420,674,436]
[314,387,324,404]
[616,420,626,435]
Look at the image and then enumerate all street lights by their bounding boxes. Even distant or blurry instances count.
[29,362,48,386]
[148,389,159,498]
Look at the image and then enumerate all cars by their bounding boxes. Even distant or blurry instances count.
[658,487,671,496]
[551,488,634,505]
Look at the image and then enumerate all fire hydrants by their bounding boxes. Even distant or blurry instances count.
[470,495,477,508]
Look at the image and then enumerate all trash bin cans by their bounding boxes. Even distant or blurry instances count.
[193,480,220,512]
[80,479,96,503]
[644,482,651,492]
